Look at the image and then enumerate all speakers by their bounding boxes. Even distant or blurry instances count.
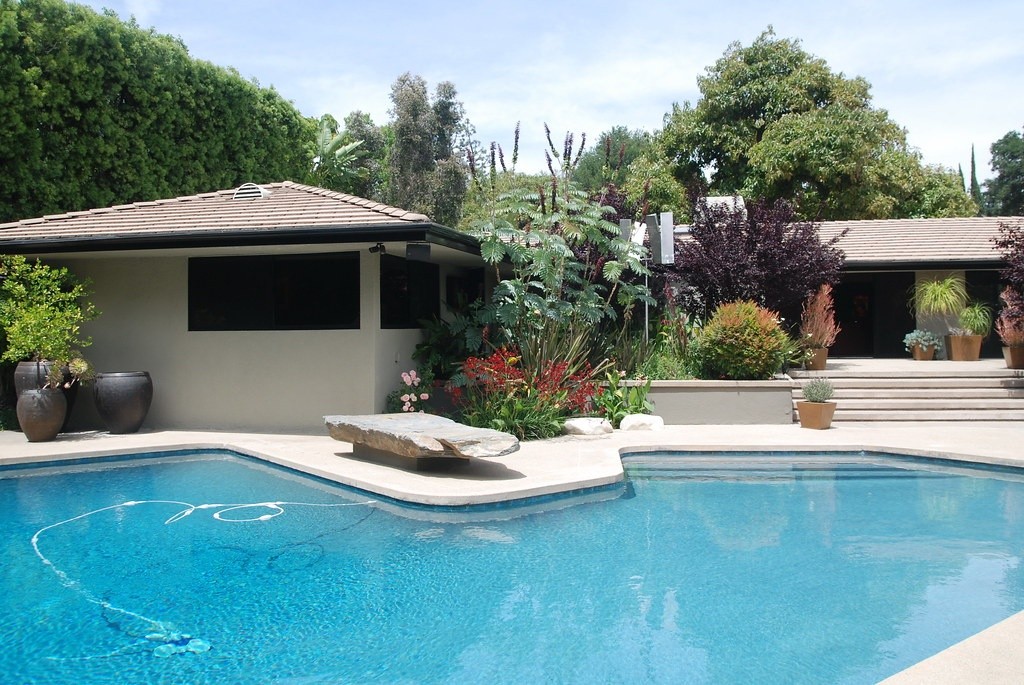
[406,244,431,260]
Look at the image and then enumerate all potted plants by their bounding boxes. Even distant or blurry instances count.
[0,253,104,444]
[902,271,991,360]
[993,285,1024,369]
[798,283,842,370]
[797,376,837,430]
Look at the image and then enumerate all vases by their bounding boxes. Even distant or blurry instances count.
[93,369,153,434]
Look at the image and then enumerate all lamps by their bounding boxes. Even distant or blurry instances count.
[369,242,386,254]
